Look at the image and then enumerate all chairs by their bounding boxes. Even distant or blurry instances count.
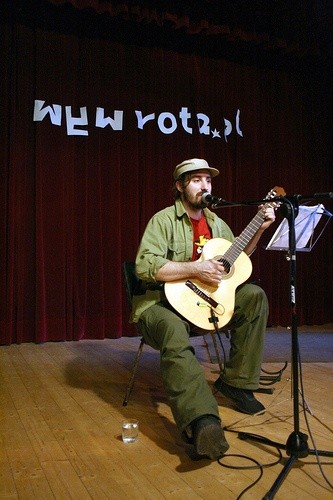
[122,261,223,407]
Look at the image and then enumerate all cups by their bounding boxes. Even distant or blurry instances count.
[122,419,138,443]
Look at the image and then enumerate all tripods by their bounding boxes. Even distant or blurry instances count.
[211,191,333,500]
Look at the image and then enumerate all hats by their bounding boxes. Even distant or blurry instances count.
[172,158,219,180]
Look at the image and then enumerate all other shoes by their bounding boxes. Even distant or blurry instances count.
[195,418,229,460]
[215,377,266,416]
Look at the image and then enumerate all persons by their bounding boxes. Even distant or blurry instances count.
[129,158,275,460]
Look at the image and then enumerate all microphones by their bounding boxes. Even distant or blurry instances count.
[201,192,231,205]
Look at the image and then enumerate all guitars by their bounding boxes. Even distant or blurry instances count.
[160,186,287,336]
